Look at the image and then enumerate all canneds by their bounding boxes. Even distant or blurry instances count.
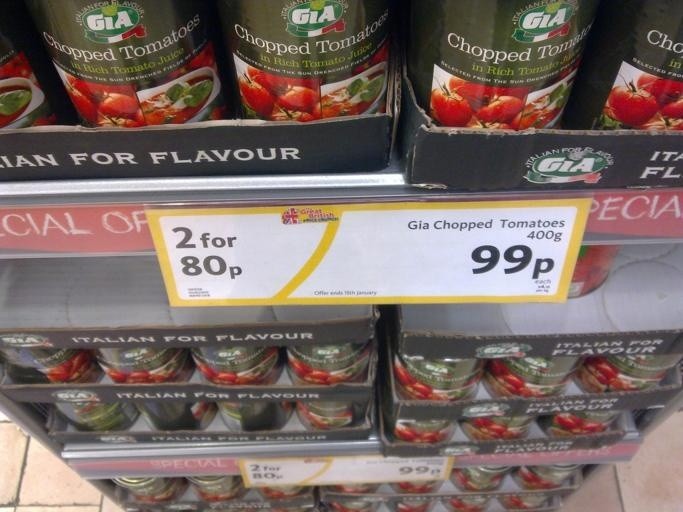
[568,245,620,299]
[0,345,683,512]
[0,0,683,131]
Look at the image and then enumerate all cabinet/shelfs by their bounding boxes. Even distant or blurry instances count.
[0,148,683,512]
